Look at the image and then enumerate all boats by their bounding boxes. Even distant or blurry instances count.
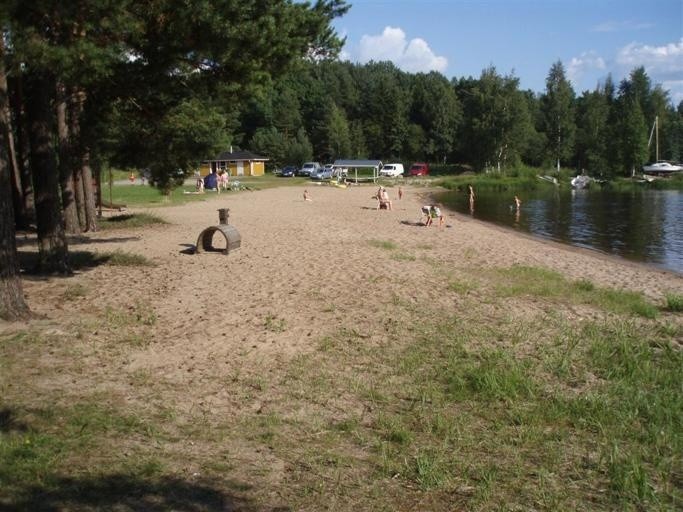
[569,173,590,190]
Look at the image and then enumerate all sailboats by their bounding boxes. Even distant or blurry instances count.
[639,114,682,177]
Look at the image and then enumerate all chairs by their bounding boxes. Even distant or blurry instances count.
[421,208,443,227]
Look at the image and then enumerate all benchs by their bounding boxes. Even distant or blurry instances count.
[376,195,389,211]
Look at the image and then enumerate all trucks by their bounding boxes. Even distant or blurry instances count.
[378,163,405,178]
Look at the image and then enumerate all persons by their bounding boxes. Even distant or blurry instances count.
[423,206,444,227]
[513,195,523,209]
[183,170,228,195]
[467,183,474,210]
[377,184,402,211]
[303,189,312,201]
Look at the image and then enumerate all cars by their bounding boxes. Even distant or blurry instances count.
[275,159,350,180]
[407,163,429,177]
